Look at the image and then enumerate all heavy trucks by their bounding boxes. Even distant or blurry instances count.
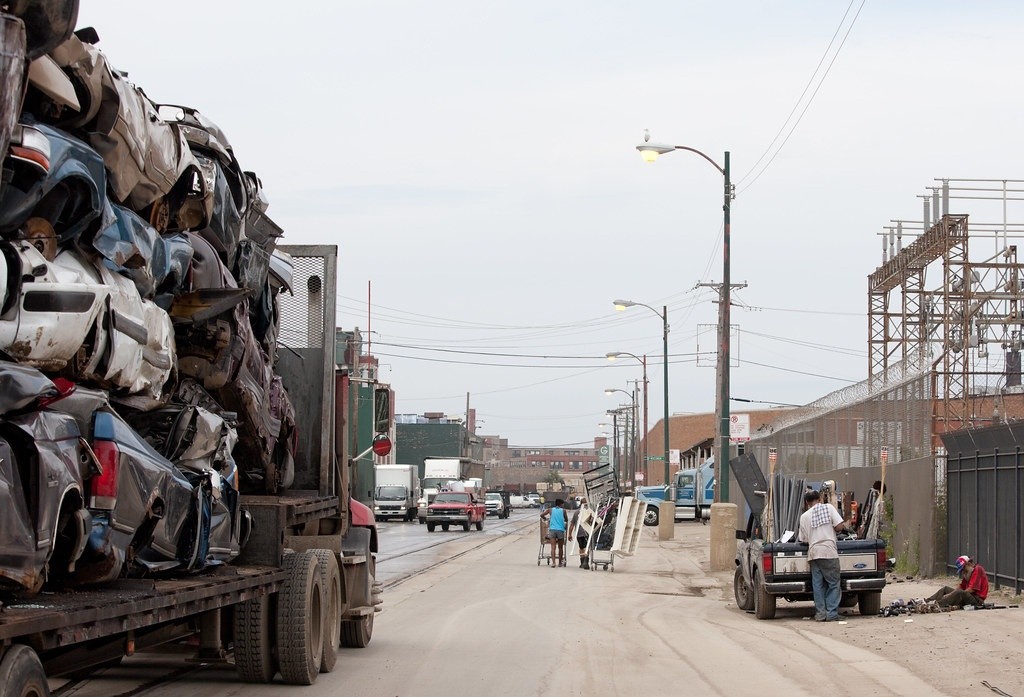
[0,244,391,696]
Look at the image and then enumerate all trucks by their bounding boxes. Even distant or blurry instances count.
[416,456,485,525]
[373,464,421,522]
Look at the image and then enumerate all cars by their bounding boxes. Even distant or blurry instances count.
[1,0,296,601]
[484,492,540,519]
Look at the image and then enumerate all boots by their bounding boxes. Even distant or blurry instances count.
[580,558,585,568]
[583,557,589,569]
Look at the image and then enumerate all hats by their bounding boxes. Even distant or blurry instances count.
[955,555,972,574]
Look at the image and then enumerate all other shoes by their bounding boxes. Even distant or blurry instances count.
[558,560,564,567]
[551,564,555,568]
[826,615,846,621]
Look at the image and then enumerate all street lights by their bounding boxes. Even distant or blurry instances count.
[613,299,675,543]
[606,387,634,495]
[605,351,648,486]
[636,139,738,574]
[598,421,621,488]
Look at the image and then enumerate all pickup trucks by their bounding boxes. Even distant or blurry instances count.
[733,499,887,620]
[635,468,716,527]
[426,491,486,532]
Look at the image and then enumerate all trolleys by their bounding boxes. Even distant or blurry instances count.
[590,550,615,572]
[538,518,568,567]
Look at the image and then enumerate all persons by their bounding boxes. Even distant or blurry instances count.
[908,555,988,611]
[799,491,848,622]
[540,499,568,568]
[568,498,591,570]
